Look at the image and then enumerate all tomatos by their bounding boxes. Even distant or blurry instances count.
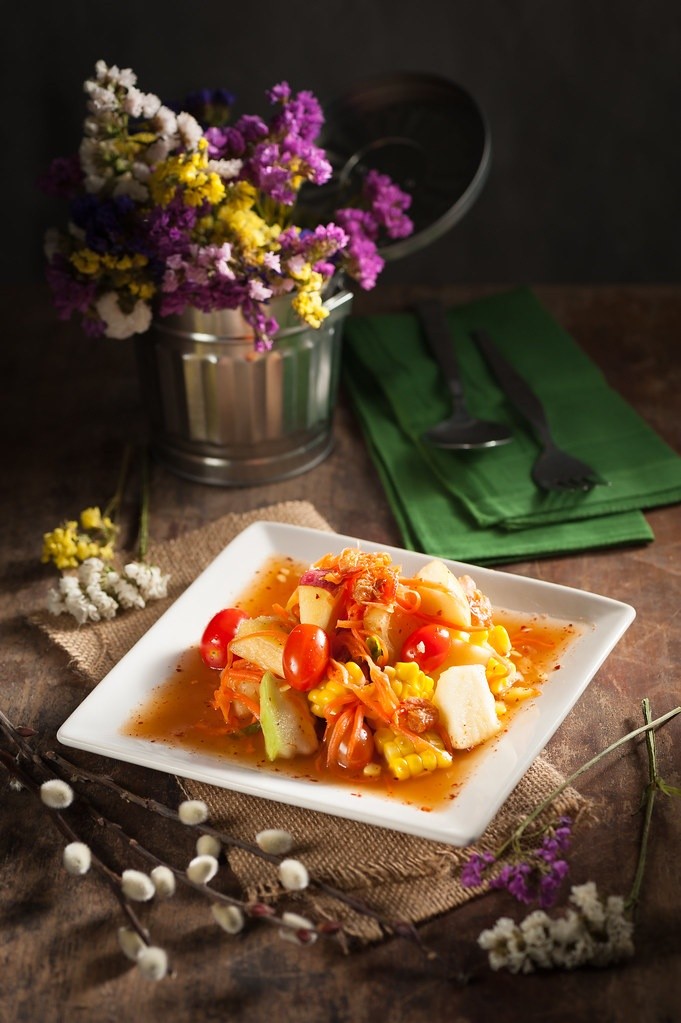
[324,716,376,769]
[281,623,328,692]
[401,625,453,674]
[201,609,247,669]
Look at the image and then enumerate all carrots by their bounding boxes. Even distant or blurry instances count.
[215,549,490,767]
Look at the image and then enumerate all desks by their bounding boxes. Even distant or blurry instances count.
[0,276,681,1023]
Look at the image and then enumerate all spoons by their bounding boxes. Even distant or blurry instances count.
[476,338,611,495]
[413,294,516,450]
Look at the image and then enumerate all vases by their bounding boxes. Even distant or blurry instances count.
[133,265,356,490]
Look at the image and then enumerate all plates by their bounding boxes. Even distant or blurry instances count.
[56,520,636,844]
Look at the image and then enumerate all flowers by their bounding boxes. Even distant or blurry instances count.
[458,702,681,975]
[40,411,172,625]
[40,58,412,364]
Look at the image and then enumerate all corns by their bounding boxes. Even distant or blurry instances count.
[377,660,434,706]
[372,725,454,778]
[307,662,365,718]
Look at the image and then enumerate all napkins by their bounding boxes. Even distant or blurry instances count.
[343,284,681,533]
[342,342,655,566]
